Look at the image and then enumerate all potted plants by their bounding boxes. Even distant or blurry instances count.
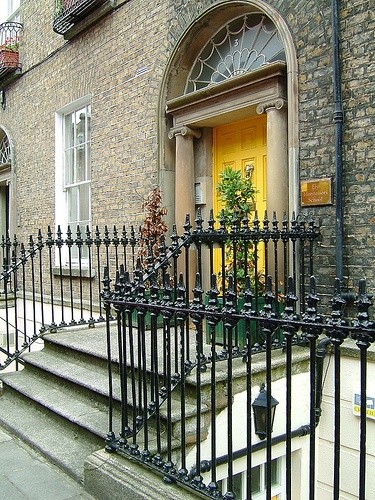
[123,187,179,329]
[205,167,286,351]
[54,0,72,22]
[0,42,19,69]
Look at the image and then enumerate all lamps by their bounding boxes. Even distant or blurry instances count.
[252,383,280,441]
